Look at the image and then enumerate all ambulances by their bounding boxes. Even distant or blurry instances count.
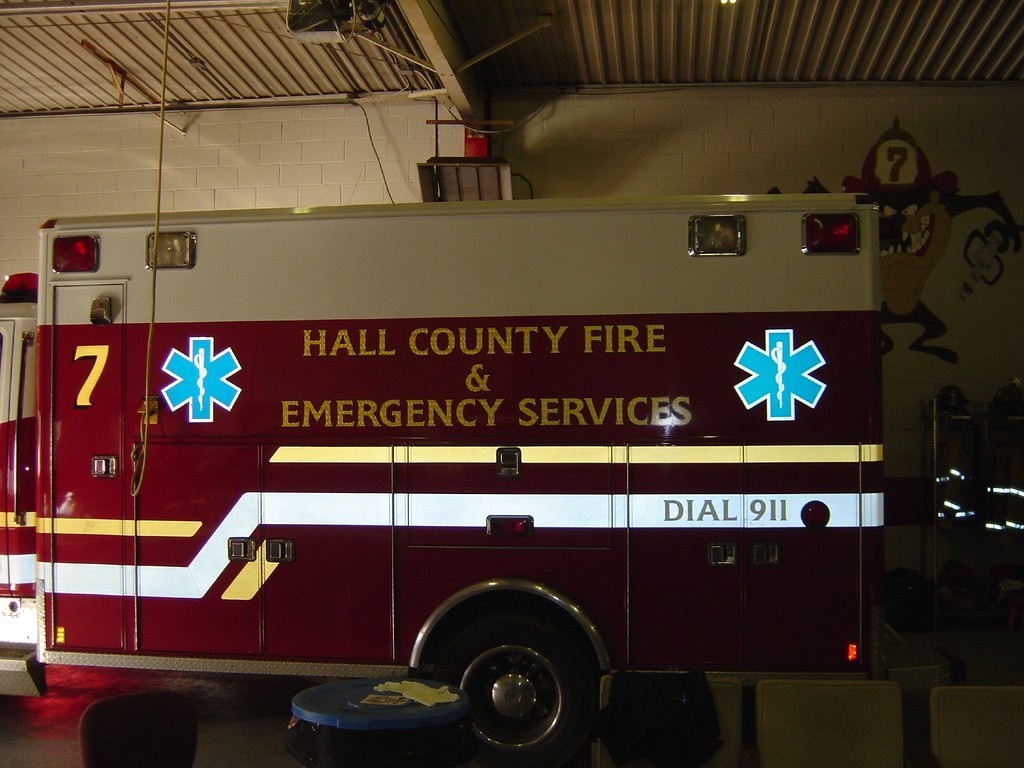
[0,192,883,768]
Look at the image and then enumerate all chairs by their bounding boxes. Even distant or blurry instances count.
[919,686,1024,768]
[743,679,919,768]
[589,675,743,768]
[79,690,198,768]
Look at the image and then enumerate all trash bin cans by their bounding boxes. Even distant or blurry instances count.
[291,677,473,768]
